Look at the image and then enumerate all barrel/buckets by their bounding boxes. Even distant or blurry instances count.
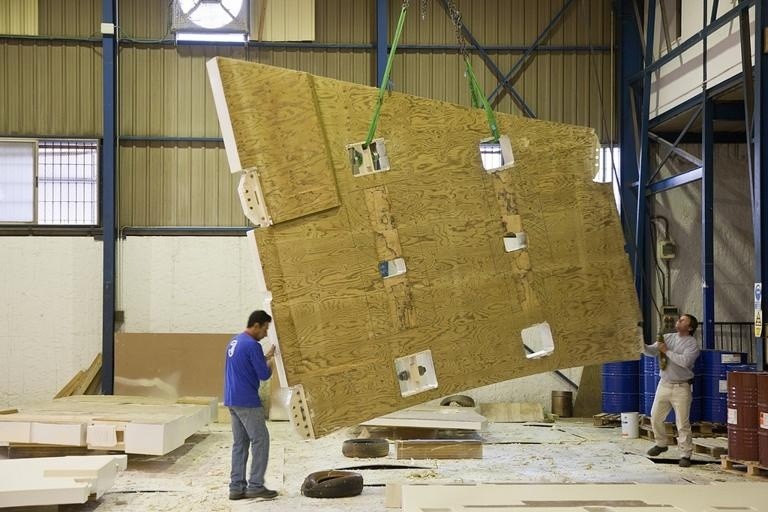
[689,349,708,423]
[756,372,768,467]
[602,361,638,413]
[551,390,573,417]
[621,412,641,438]
[706,348,747,429]
[642,356,662,416]
[727,370,756,460]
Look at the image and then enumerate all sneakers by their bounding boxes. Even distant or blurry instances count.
[679,456,691,467]
[245,487,278,499]
[229,488,249,500]
[647,445,668,456]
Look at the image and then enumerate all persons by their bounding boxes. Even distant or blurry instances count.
[639,315,700,468]
[223,310,279,501]
